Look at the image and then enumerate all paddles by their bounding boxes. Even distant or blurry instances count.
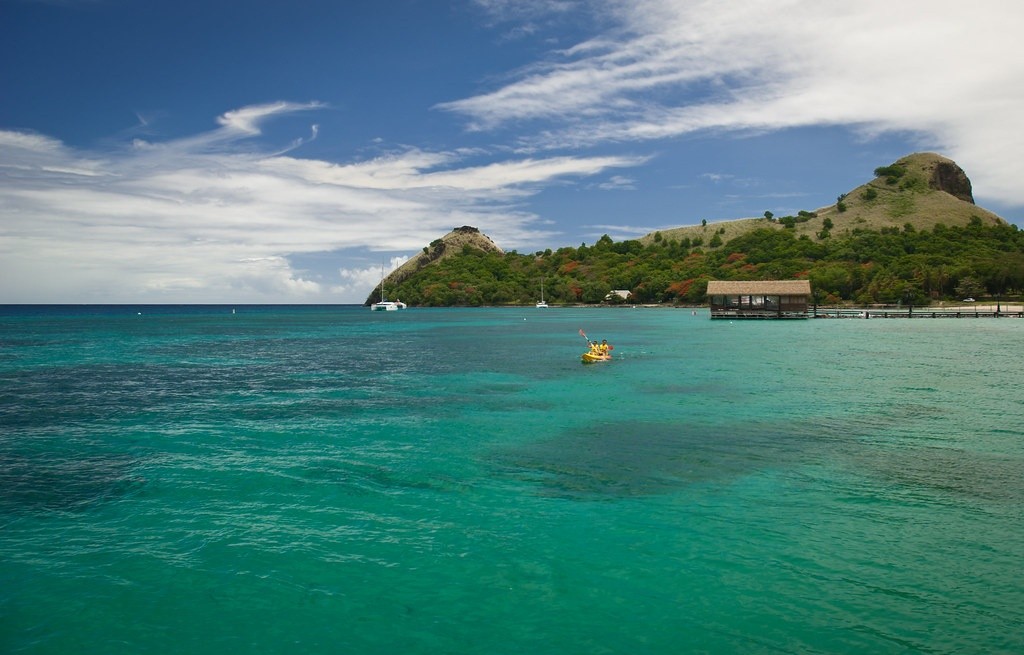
[604,345,614,352]
[579,329,596,352]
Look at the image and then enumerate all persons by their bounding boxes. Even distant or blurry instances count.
[587,341,599,355]
[599,340,608,356]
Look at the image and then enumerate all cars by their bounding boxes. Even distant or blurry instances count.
[963,298,976,303]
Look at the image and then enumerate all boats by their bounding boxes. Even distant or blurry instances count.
[535,300,549,309]
[580,353,612,364]
[370,262,407,314]
